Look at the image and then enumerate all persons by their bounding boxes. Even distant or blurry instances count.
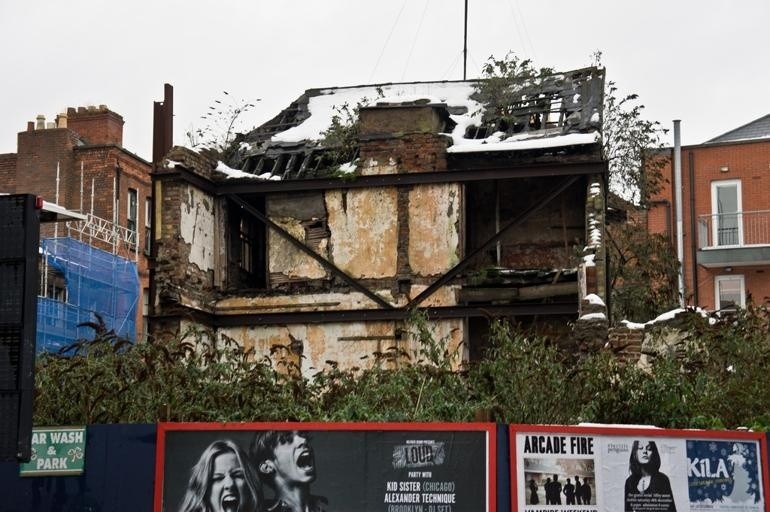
[527,471,592,505]
[724,442,753,502]
[620,440,677,511]
[175,438,264,512]
[247,428,329,511]
